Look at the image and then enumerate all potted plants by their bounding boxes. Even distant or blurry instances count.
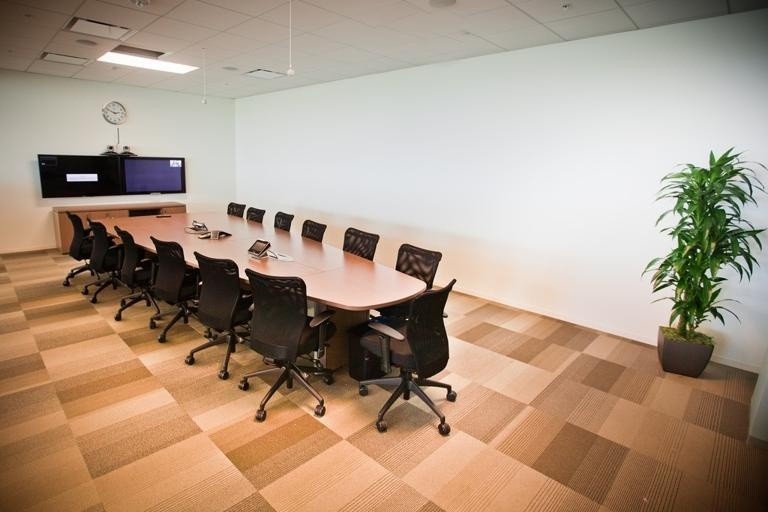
[642,148,768,377]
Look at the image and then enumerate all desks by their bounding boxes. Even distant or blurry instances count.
[52,201,186,255]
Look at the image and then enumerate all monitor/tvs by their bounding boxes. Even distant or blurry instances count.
[123,155,186,195]
[38,153,124,199]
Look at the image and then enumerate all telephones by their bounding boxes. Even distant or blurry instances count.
[199,230,232,240]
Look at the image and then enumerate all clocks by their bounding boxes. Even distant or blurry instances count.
[103,100,127,124]
